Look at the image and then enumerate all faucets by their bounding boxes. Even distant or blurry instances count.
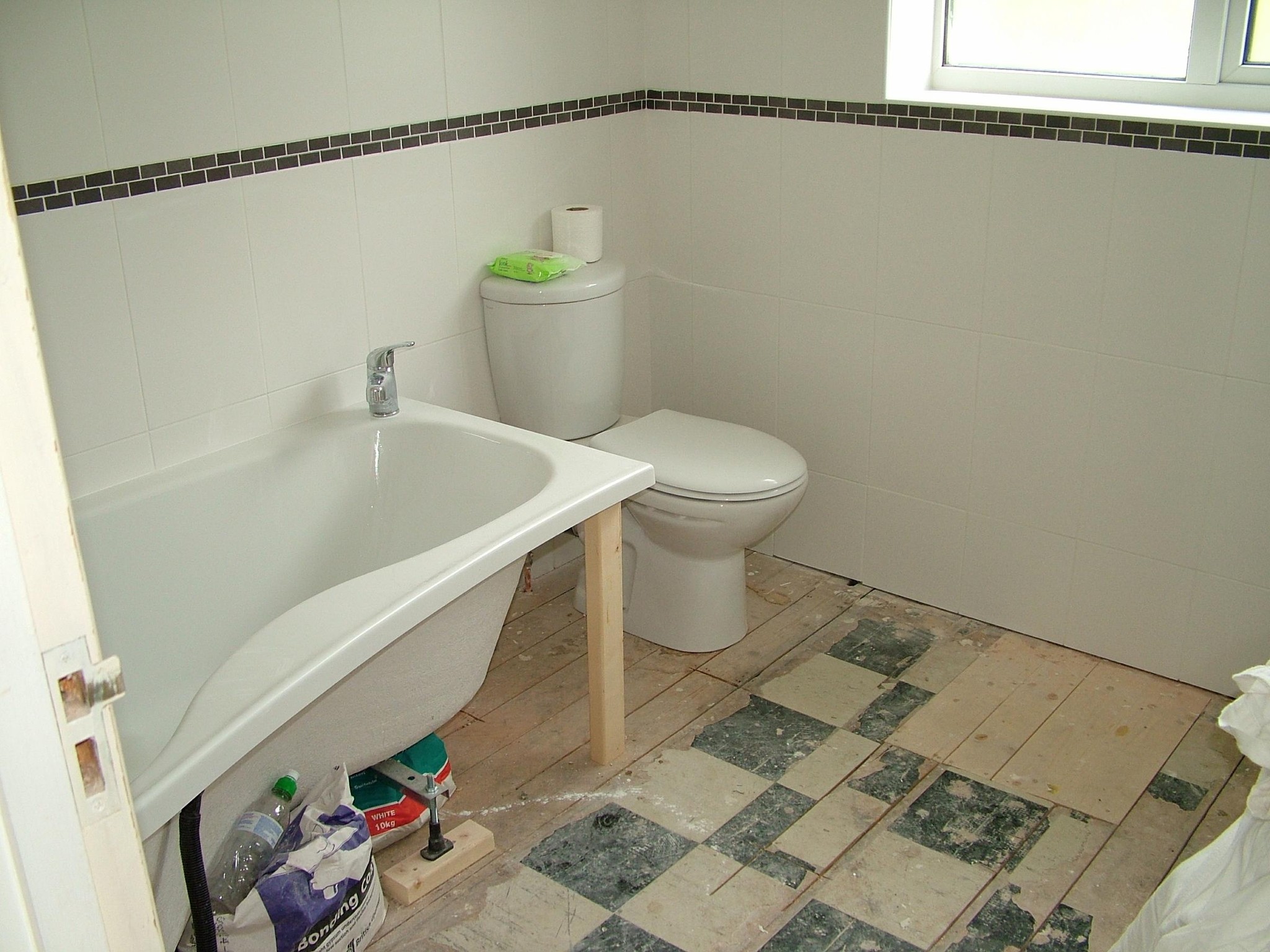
[365,342,417,418]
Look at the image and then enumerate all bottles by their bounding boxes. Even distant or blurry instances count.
[207,770,300,916]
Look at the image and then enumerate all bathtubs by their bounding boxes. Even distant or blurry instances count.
[71,395,657,952]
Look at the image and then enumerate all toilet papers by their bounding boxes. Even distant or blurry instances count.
[551,202,603,262]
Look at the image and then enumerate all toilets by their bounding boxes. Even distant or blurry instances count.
[479,258,811,654]
